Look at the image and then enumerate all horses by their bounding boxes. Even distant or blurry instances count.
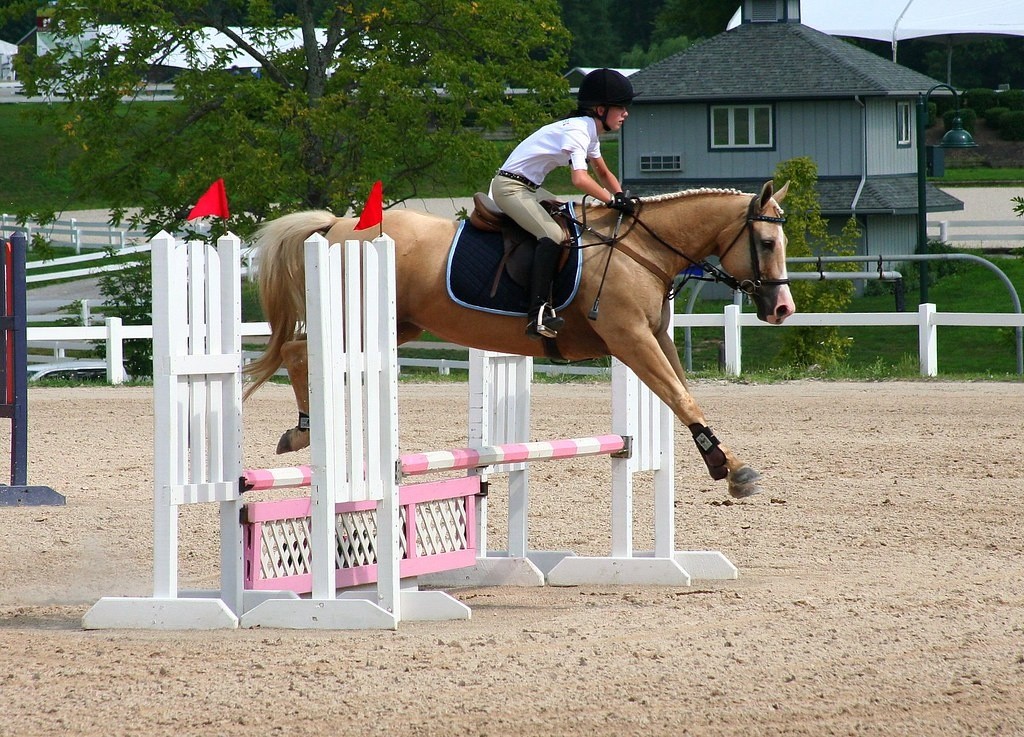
[241,178,798,499]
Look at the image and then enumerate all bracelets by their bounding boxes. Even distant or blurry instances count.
[609,193,616,208]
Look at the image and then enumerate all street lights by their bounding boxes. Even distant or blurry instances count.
[915,82,980,304]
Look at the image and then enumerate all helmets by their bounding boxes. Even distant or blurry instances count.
[578,68,632,106]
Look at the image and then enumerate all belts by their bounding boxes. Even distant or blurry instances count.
[498,170,541,189]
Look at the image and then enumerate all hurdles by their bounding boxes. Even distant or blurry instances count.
[0,230,67,508]
[81,231,740,629]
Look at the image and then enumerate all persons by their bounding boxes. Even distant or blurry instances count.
[492,65,636,340]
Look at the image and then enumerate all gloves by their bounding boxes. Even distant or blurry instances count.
[608,192,635,214]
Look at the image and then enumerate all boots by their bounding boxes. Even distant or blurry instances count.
[525,237,565,337]
